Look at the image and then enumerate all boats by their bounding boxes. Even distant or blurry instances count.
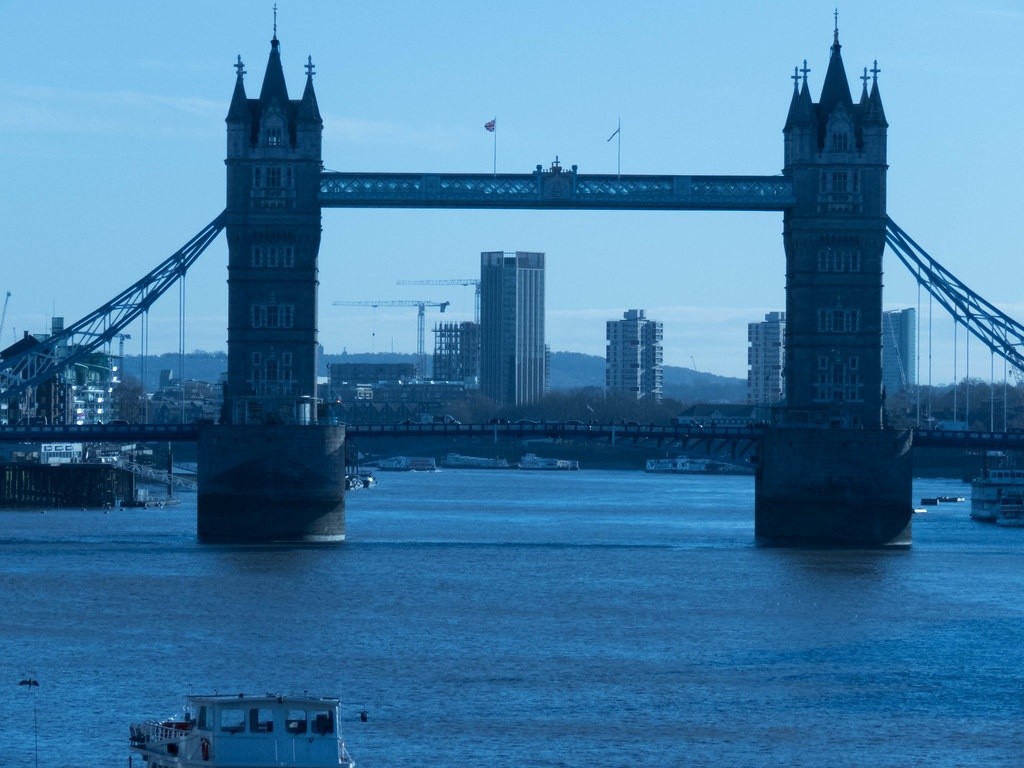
[646,456,713,474]
[377,456,437,472]
[518,453,579,471]
[969,467,1024,521]
[997,490,1024,528]
[128,690,355,768]
[442,452,509,469]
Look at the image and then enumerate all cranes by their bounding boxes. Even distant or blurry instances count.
[330,299,450,378]
[395,278,481,379]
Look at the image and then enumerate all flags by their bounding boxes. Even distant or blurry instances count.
[485,119,495,133]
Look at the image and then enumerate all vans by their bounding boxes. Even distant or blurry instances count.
[15,415,48,426]
[432,414,462,424]
[671,416,703,428]
[400,414,430,424]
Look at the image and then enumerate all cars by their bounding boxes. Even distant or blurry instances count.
[565,420,592,431]
[489,418,511,424]
[514,420,539,424]
[107,421,130,424]
[628,421,643,427]
